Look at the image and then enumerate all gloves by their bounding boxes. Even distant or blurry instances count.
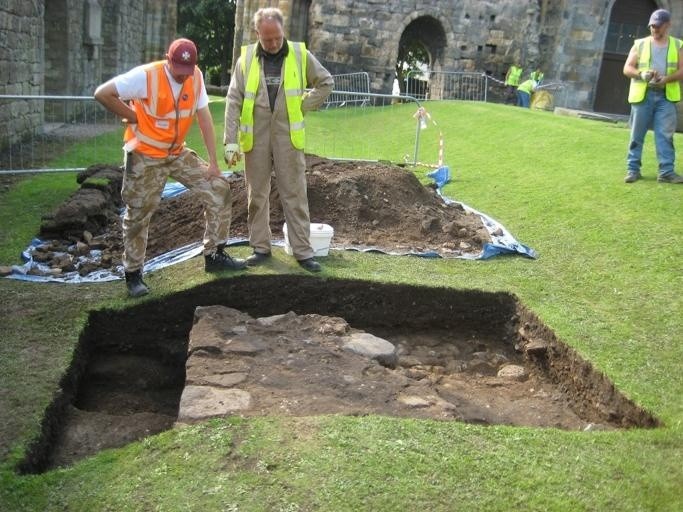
[222,143,241,169]
[639,69,663,86]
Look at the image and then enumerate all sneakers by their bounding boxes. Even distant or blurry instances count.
[656,173,682,186]
[623,171,642,184]
[200,246,248,273]
[122,267,149,299]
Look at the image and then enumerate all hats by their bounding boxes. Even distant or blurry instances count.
[165,37,197,76]
[647,8,672,28]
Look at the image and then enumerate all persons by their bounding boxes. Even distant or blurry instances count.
[94,38,248,296]
[222,7,335,271]
[623,9,683,184]
[504,60,545,109]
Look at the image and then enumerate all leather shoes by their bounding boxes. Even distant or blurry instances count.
[245,251,272,266]
[297,257,322,273]
